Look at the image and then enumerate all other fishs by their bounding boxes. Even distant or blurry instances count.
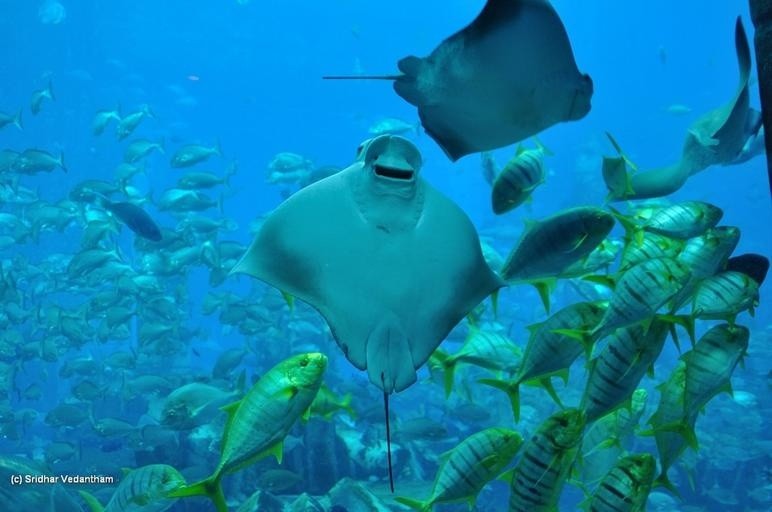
[0,0,772,512]
